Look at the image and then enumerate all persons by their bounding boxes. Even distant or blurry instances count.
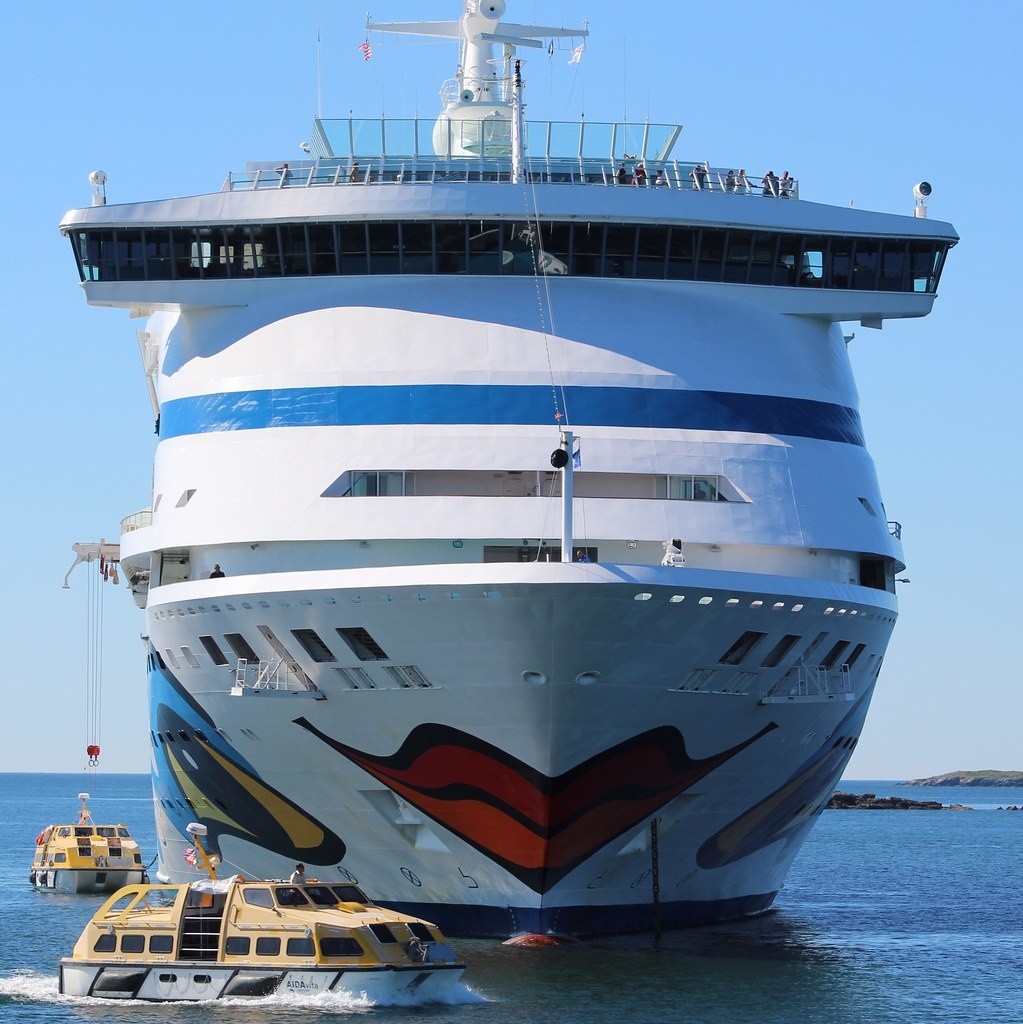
[632,163,647,186]
[577,550,591,563]
[209,564,224,578]
[653,170,668,186]
[690,483,706,498]
[290,863,306,893]
[617,163,627,184]
[779,171,793,199]
[77,829,85,856]
[725,170,735,194]
[733,168,757,195]
[761,171,779,198]
[689,165,707,190]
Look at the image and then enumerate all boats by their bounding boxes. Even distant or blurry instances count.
[57,0,963,940]
[57,882,465,1000]
[29,823,150,893]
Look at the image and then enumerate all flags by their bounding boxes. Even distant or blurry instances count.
[182,847,198,868]
[572,449,581,469]
